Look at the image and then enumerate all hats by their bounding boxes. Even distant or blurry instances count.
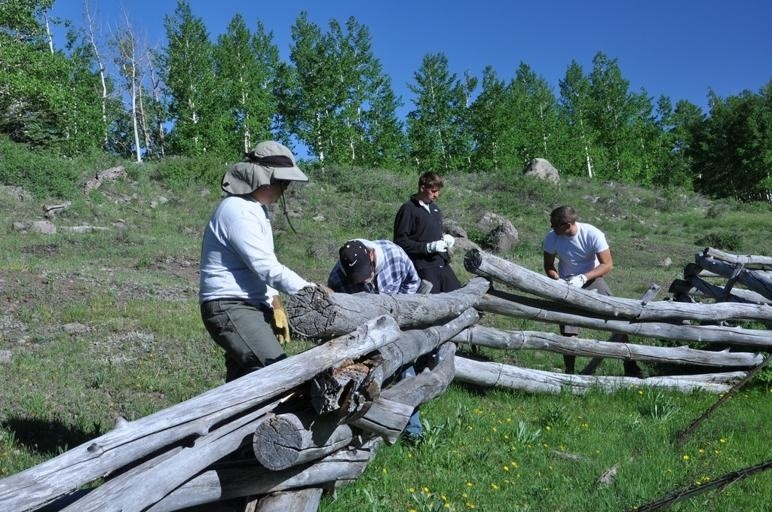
[339,240,372,284]
[221,141,310,197]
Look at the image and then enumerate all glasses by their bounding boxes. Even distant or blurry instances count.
[550,222,570,229]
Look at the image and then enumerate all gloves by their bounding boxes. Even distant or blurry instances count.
[554,273,588,288]
[426,233,455,254]
[273,309,291,344]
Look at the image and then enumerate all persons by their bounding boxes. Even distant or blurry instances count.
[392,171,461,295]
[541,205,644,378]
[327,238,426,445]
[197,140,338,460]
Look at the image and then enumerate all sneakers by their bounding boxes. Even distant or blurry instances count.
[401,434,422,447]
[622,363,650,378]
